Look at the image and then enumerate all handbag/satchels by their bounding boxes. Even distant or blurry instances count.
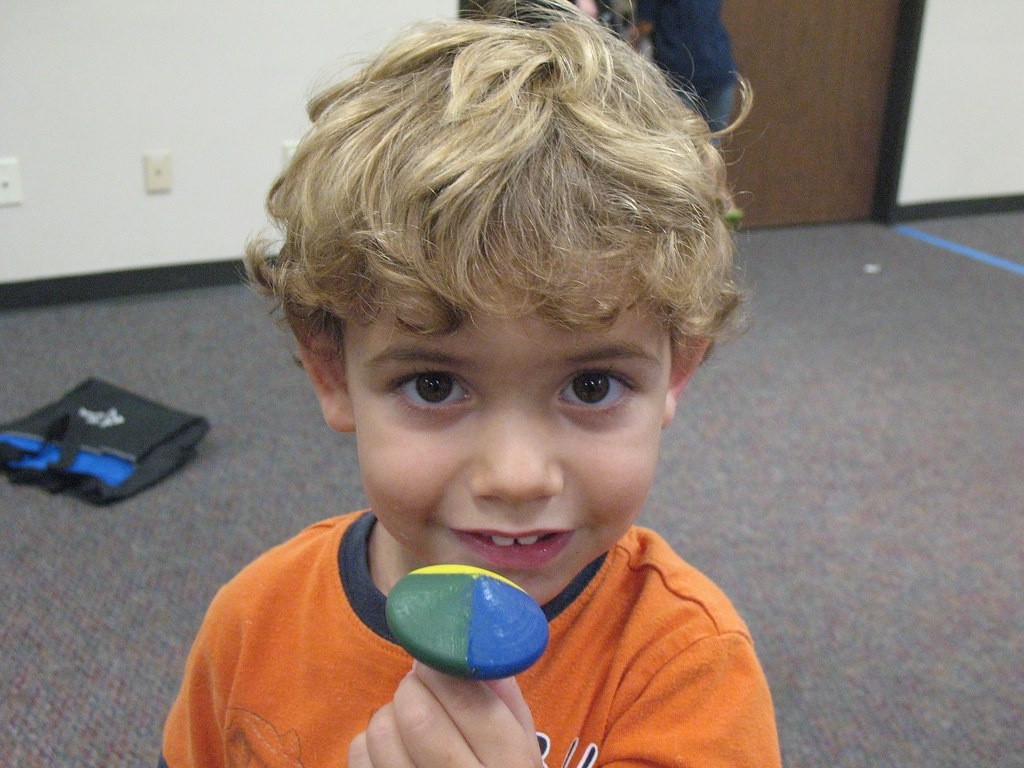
[0,378,211,506]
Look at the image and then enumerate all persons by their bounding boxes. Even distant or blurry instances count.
[157,0,784,768]
